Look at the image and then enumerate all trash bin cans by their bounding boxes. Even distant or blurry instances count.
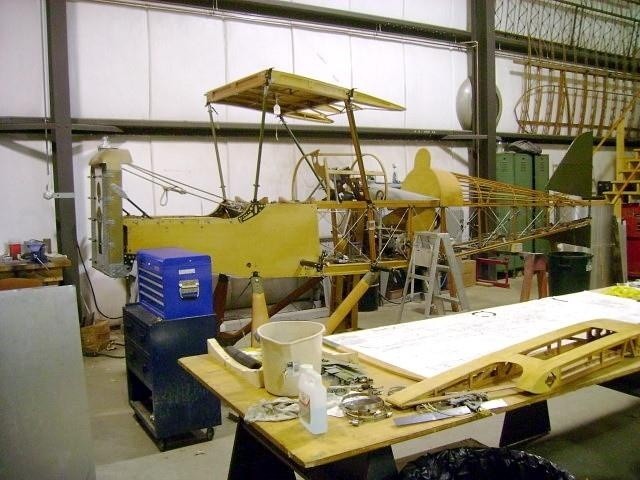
[395,448,578,480]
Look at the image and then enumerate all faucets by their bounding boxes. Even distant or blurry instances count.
[316,249,328,272]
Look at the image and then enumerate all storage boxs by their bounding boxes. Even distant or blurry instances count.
[447,259,478,290]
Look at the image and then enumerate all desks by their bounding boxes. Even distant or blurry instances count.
[176,280,640,479]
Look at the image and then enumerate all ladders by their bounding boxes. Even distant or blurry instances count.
[397,231,468,321]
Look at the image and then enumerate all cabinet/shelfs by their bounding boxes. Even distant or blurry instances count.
[0,253,72,287]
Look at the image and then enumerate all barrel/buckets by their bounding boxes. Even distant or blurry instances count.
[257,321,324,396]
[554,199,615,288]
[298,365,328,433]
